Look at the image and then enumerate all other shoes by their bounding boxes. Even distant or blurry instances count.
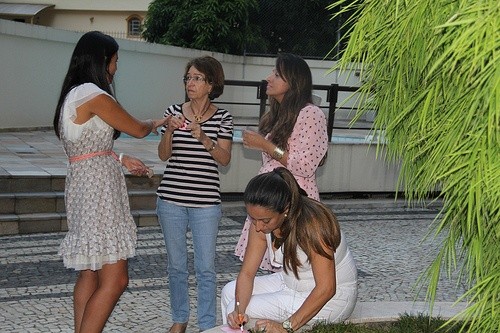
[166,321,188,333]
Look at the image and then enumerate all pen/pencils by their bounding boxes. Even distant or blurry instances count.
[235,296,244,332]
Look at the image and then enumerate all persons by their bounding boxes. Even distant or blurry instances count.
[234,52,328,272]
[53,31,172,333]
[221,166,358,333]
[154,55,233,333]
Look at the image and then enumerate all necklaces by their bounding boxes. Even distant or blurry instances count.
[190,102,211,122]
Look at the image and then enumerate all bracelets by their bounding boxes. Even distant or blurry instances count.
[273,144,286,160]
[119,153,124,163]
[208,141,215,152]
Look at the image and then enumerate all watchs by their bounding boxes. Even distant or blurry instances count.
[282,320,294,333]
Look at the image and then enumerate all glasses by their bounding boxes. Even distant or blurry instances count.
[182,72,209,85]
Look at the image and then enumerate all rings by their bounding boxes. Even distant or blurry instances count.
[261,326,266,333]
[228,321,232,326]
